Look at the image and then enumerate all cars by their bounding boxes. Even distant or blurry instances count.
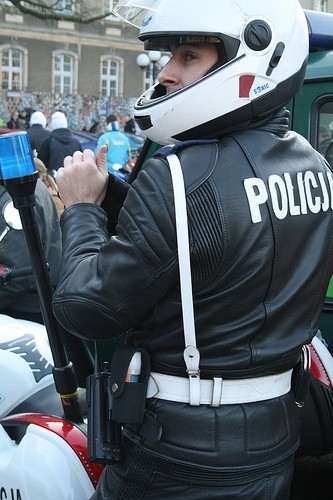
[285,9,333,350]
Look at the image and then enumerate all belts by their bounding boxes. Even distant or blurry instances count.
[145,371,294,407]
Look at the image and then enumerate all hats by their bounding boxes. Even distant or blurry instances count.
[51,111,67,129]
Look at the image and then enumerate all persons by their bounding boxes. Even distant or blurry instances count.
[124,115,136,135]
[0,158,65,326]
[51,0,333,500]
[318,125,333,159]
[94,113,132,183]
[37,111,83,207]
[0,104,36,131]
[25,111,53,158]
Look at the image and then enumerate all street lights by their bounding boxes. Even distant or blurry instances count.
[136,50,172,85]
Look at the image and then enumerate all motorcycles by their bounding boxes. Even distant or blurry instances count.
[1,129,103,500]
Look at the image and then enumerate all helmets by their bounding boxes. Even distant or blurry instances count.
[112,0,311,148]
[29,112,46,128]
